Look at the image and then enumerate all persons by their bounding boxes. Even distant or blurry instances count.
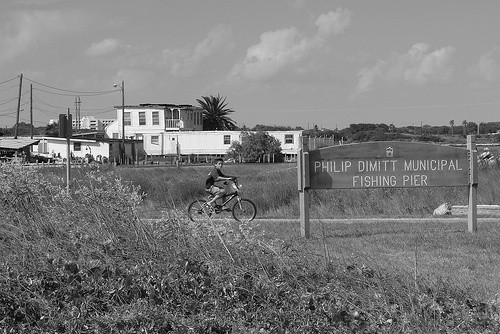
[479,148,491,167]
[205,159,234,211]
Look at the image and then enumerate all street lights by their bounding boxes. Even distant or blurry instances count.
[112,81,125,165]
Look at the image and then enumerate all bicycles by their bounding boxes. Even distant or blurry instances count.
[187,176,257,223]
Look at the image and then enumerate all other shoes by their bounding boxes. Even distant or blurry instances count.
[222,205,230,210]
[206,202,213,208]
